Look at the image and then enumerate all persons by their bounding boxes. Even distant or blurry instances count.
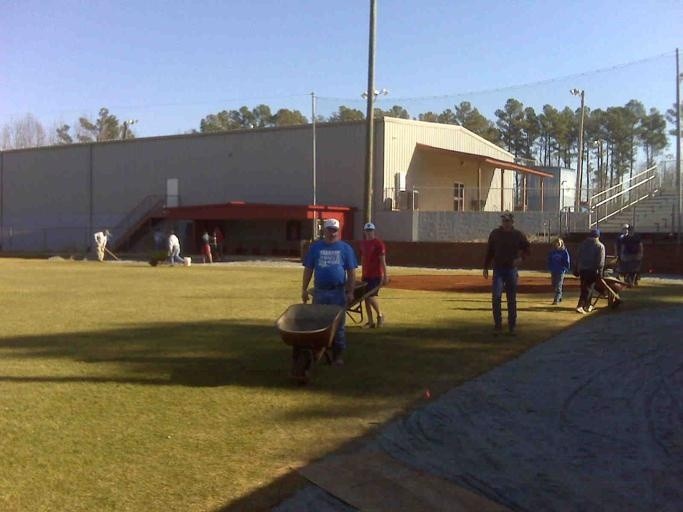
[212,227,224,260]
[613,223,644,285]
[546,238,570,305]
[199,231,213,263]
[168,232,186,266]
[572,228,606,314]
[301,219,357,368]
[358,223,388,326]
[482,210,531,332]
[94,229,109,261]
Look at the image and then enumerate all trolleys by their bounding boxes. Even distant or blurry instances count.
[574,269,630,313]
[273,289,351,386]
[305,272,391,329]
[615,255,644,288]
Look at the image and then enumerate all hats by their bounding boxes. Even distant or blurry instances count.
[364,222,375,231]
[500,209,515,220]
[592,229,600,237]
[324,218,339,231]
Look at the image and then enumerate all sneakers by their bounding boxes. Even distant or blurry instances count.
[585,304,594,312]
[575,306,587,314]
[361,323,375,329]
[377,313,385,328]
[332,351,345,369]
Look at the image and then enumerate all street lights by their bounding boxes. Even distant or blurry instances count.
[360,87,389,120]
[568,87,585,211]
[120,117,138,141]
